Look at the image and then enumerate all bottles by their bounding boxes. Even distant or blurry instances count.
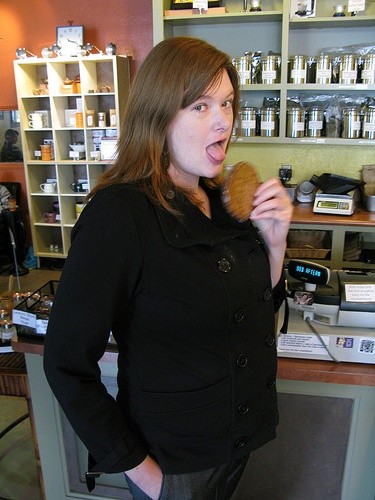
[0,320,15,346]
[0,310,11,321]
[87,110,106,127]
[232,51,375,140]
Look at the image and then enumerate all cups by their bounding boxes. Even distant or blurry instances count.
[40,183,56,194]
[28,113,43,129]
[44,212,56,223]
[76,113,84,128]
[40,145,51,161]
[72,182,86,192]
[367,196,375,213]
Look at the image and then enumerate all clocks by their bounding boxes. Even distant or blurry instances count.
[55,18,85,56]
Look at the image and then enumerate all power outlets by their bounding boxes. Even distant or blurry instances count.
[282,165,291,180]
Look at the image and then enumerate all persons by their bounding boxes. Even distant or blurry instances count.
[43,36,296,500]
[0,185,29,276]
[0,129,23,162]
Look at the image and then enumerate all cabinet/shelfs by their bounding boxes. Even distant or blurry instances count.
[12,55,132,269]
[283,200,375,270]
[11,333,375,500]
[151,0,375,146]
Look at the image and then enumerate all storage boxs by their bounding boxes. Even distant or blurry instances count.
[64,109,77,127]
[92,129,118,151]
[286,184,298,203]
[76,97,83,112]
[46,178,57,191]
[75,203,87,220]
[109,108,117,127]
[359,187,375,212]
[62,85,77,94]
[12,280,60,344]
[34,110,51,128]
